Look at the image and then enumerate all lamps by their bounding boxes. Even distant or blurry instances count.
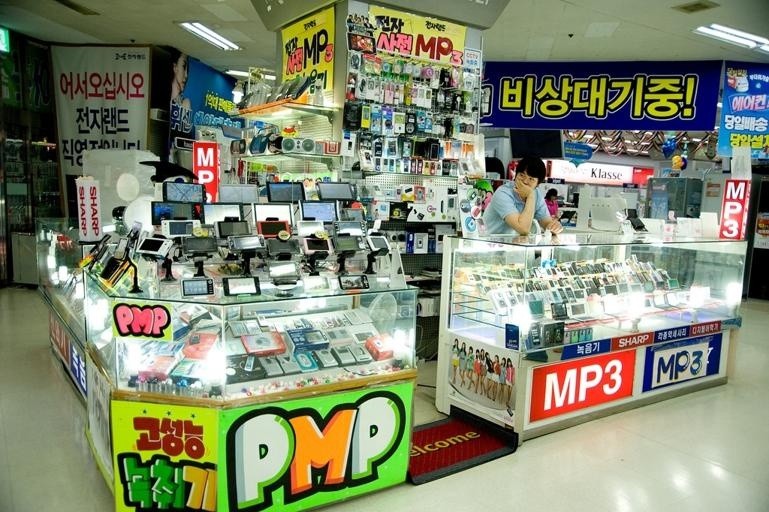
[191,20,243,53]
[173,22,232,53]
[759,45,769,53]
[223,68,276,82]
[692,25,757,49]
[710,22,769,46]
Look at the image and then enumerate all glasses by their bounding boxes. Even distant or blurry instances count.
[519,173,537,184]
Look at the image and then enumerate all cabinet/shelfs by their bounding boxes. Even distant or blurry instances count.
[82,265,421,512]
[35,216,121,409]
[435,230,749,447]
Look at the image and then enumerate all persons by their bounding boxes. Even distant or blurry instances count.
[170,53,194,125]
[482,155,564,235]
[544,188,559,218]
[451,339,515,409]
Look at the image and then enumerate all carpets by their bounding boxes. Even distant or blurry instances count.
[405,404,518,487]
